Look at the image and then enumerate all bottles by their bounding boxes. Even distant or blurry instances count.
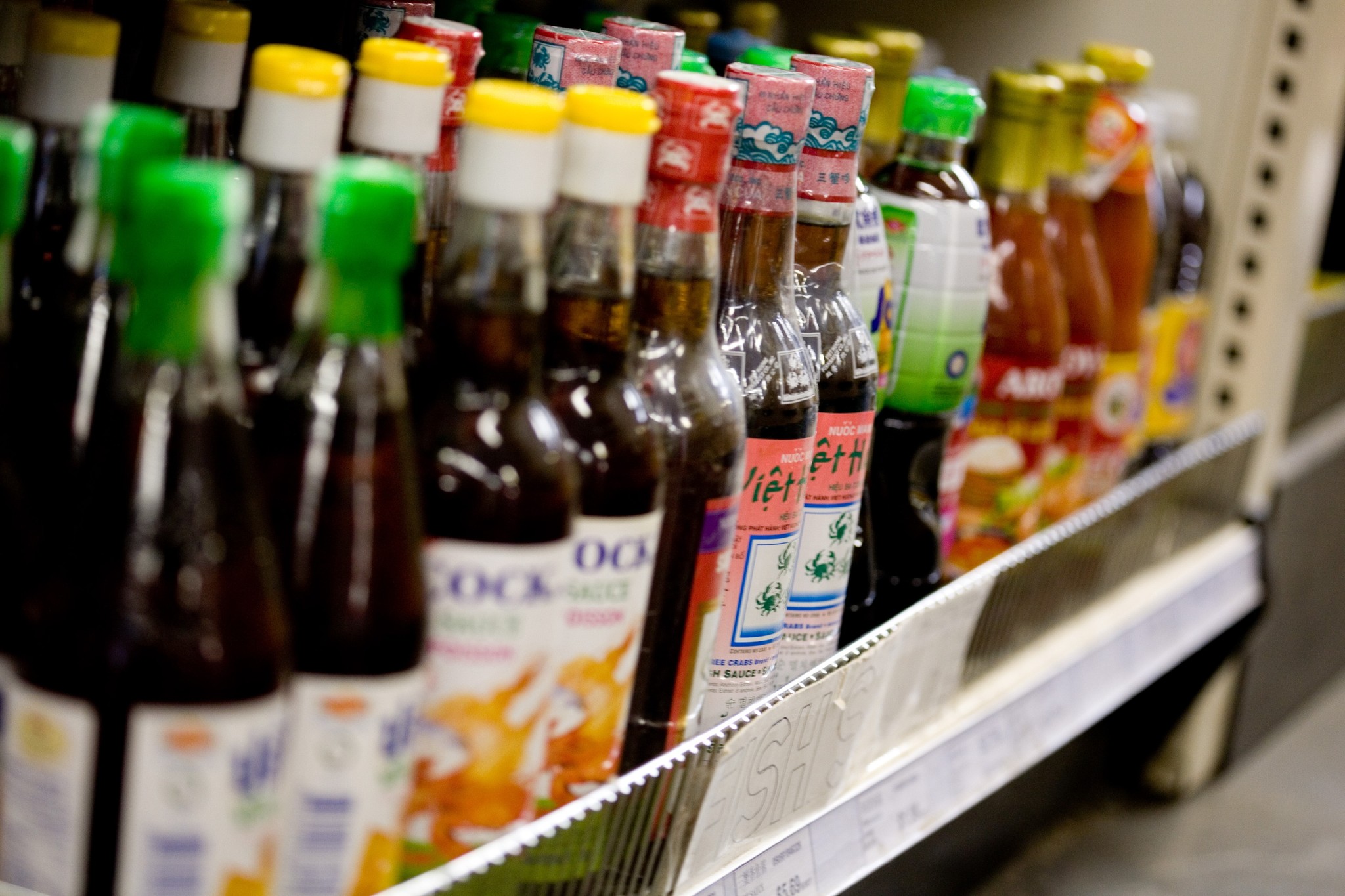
[0,1,993,896]
[939,42,1210,574]
